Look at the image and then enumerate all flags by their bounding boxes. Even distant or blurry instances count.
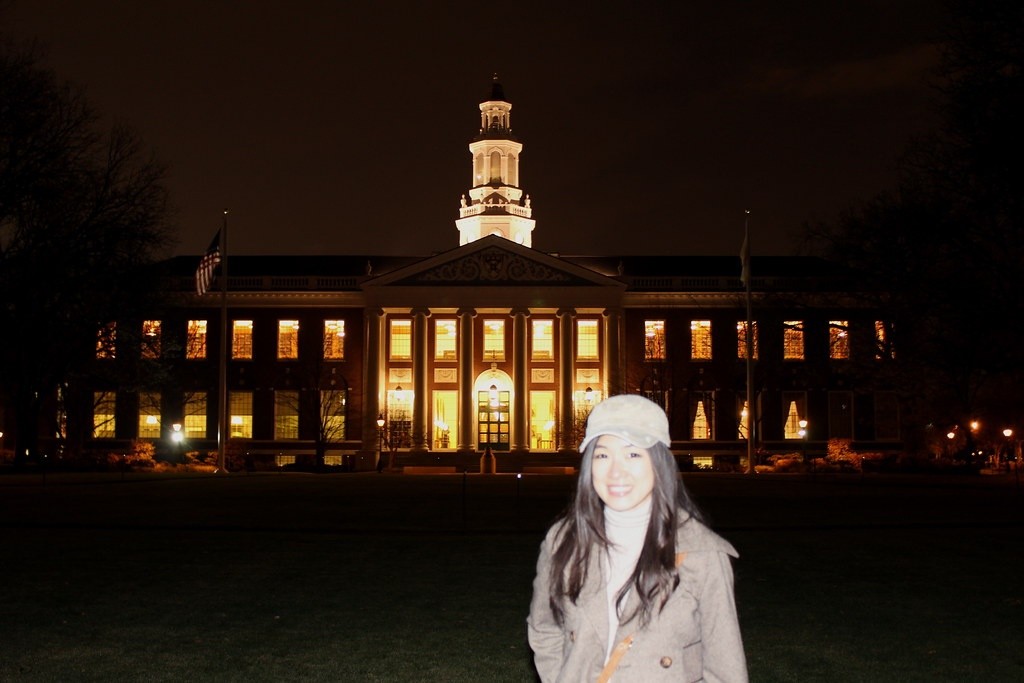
[195,226,222,299]
[738,230,748,291]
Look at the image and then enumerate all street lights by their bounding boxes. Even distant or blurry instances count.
[172,419,187,466]
[376,412,386,474]
[798,414,811,465]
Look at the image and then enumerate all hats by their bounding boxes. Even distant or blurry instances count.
[578,394,671,454]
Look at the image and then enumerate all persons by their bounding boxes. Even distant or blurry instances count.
[525,393,752,683]
